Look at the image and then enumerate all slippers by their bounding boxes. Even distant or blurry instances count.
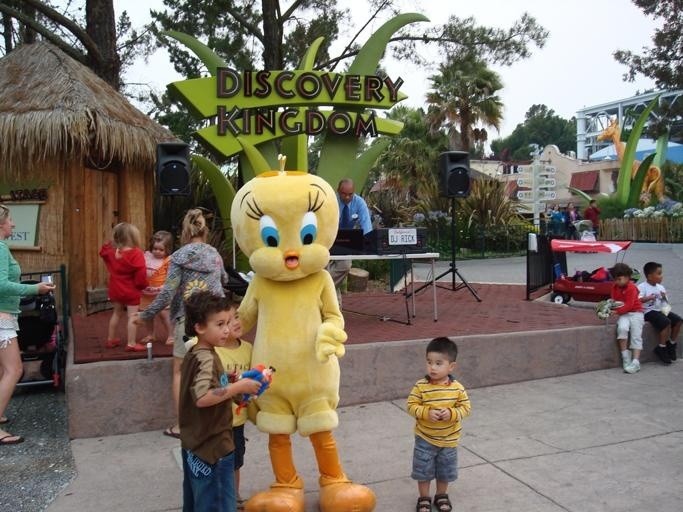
[163,425,182,438]
[164,337,175,346]
[142,335,159,344]
[0,434,25,445]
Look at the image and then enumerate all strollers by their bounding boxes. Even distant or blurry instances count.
[14,280,65,393]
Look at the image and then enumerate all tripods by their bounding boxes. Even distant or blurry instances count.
[406,198,482,302]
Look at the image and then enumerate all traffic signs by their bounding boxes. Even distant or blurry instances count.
[517,165,533,175]
[540,191,556,200]
[516,179,532,187]
[516,191,532,201]
[541,180,556,187]
[538,165,555,175]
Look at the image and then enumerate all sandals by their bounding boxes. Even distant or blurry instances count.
[433,493,452,512]
[416,497,432,512]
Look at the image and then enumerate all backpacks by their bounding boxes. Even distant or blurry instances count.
[15,278,58,352]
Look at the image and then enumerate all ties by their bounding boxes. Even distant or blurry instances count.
[341,204,351,229]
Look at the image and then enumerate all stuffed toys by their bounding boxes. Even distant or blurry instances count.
[185,154,377,512]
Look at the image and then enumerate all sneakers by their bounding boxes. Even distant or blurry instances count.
[106,338,124,347]
[653,344,671,363]
[666,340,677,361]
[624,361,641,374]
[620,350,633,371]
[125,344,147,351]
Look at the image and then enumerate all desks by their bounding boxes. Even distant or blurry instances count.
[330,252,440,323]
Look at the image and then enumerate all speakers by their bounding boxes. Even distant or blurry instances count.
[156,144,191,196]
[439,152,470,198]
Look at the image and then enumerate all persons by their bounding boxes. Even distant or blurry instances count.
[0,206,56,444]
[407,337,471,512]
[140,231,175,345]
[178,290,261,512]
[327,179,373,312]
[636,262,683,363]
[214,298,253,510]
[99,223,149,352]
[132,210,229,439]
[610,264,644,374]
[236,365,276,415]
[547,199,601,242]
